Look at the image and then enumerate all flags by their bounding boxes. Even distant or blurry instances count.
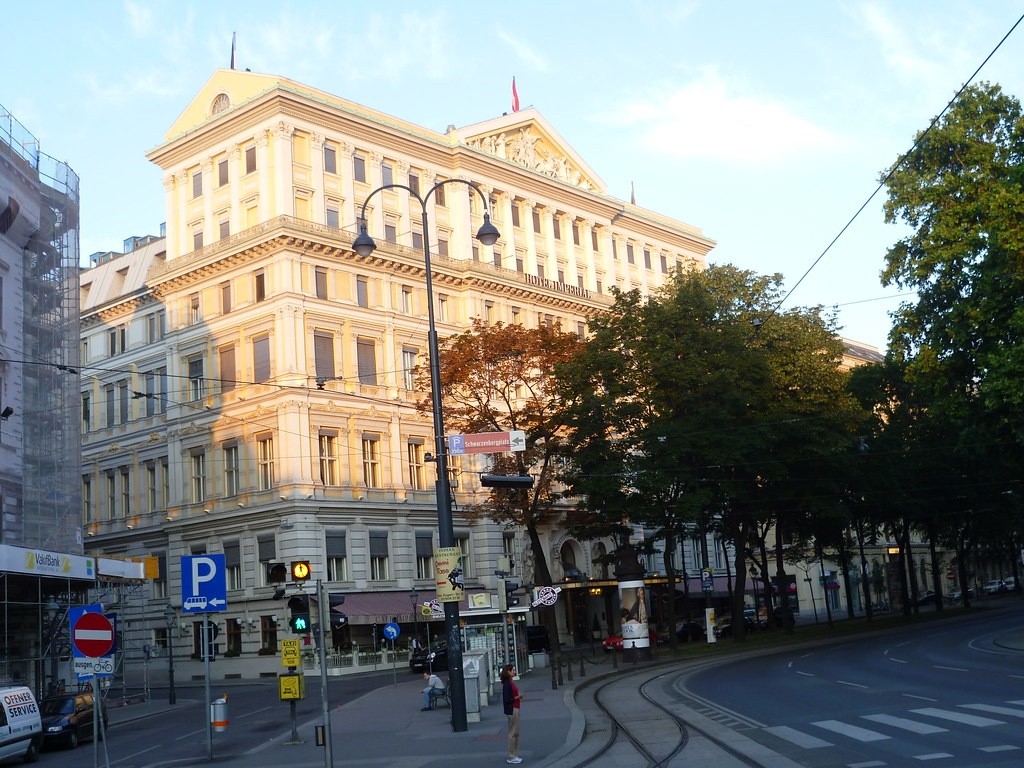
[232,32,238,71]
[513,78,519,112]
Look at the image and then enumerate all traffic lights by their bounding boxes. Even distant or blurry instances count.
[291,560,310,582]
[499,579,520,613]
[323,591,346,632]
[266,562,288,584]
[290,595,310,633]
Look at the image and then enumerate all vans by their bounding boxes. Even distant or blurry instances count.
[0,686,42,767]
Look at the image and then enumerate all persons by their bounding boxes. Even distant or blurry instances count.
[499,663,524,764]
[622,587,648,624]
[412,635,422,654]
[420,671,446,711]
[433,634,440,642]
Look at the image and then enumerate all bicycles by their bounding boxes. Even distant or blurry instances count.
[943,594,962,609]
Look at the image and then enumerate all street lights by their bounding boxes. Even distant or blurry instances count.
[409,587,420,651]
[349,180,501,731]
[748,564,762,634]
[163,603,177,705]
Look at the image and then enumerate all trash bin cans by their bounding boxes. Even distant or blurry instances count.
[211,697,229,733]
[532,652,550,668]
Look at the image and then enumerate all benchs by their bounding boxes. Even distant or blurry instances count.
[429,679,450,710]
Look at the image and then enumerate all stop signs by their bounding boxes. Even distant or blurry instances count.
[74,612,114,657]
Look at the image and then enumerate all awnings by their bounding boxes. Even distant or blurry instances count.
[664,577,765,598]
[310,589,492,625]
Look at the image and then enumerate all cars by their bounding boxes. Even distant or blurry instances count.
[603,629,658,652]
[37,691,109,754]
[410,641,447,673]
[1004,577,1015,591]
[657,606,796,647]
[869,590,936,610]
[981,580,1005,595]
[945,587,974,602]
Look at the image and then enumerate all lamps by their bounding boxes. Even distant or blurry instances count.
[246,618,256,629]
[44,594,60,610]
[272,616,280,626]
[181,623,190,633]
[237,619,245,629]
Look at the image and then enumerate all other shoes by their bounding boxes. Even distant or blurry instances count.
[507,757,523,764]
[420,707,432,711]
